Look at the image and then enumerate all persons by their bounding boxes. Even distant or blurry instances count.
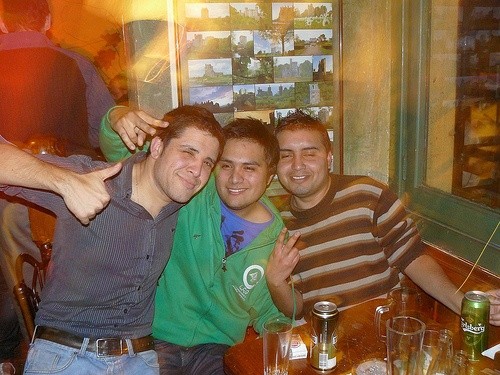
[99,105,293,375]
[264,110,500,327]
[0,0,117,347]
[0,105,225,375]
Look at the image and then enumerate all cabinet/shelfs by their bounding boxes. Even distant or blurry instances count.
[451,0,500,211]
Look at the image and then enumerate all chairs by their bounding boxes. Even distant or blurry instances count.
[13,241,52,342]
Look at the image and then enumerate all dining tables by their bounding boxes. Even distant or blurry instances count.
[224,264,500,375]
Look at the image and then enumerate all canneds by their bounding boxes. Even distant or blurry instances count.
[459,290,490,361]
[308,300,340,373]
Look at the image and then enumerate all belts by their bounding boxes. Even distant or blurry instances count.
[34,326,155,358]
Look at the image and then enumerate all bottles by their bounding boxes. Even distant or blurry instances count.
[434,331,452,375]
[449,350,468,374]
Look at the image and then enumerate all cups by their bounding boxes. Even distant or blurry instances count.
[407,330,449,375]
[375,287,424,344]
[386,316,426,375]
[262,319,293,375]
